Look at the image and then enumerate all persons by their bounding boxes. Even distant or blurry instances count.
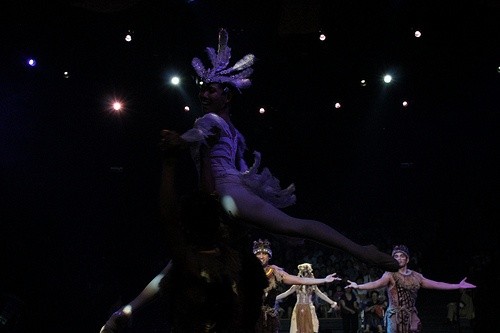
[156,129,278,333]
[98,78,402,333]
[269,258,390,333]
[275,262,339,333]
[249,238,343,332]
[343,245,476,333]
[232,249,269,309]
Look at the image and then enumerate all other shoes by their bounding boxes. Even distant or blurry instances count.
[362,245,399,272]
[99,310,131,333]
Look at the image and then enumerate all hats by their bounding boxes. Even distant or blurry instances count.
[391,245,410,258]
[253,238,273,257]
[297,263,313,275]
